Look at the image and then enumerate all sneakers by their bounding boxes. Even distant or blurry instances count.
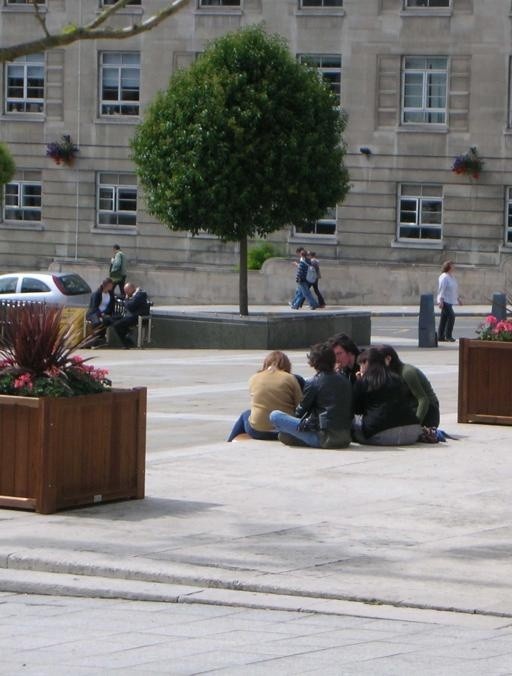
[278,432,308,447]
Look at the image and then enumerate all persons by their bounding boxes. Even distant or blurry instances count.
[327,332,364,383]
[291,250,317,310]
[114,282,150,350]
[269,343,354,450]
[86,278,116,349]
[352,348,421,448]
[227,351,304,443]
[299,251,326,308]
[380,344,440,430]
[288,247,319,308]
[436,260,465,344]
[109,244,127,295]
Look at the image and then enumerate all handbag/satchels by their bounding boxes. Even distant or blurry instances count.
[107,252,124,287]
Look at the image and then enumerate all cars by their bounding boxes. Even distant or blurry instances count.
[0,271,94,320]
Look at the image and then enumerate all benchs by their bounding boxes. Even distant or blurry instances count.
[82,297,152,350]
